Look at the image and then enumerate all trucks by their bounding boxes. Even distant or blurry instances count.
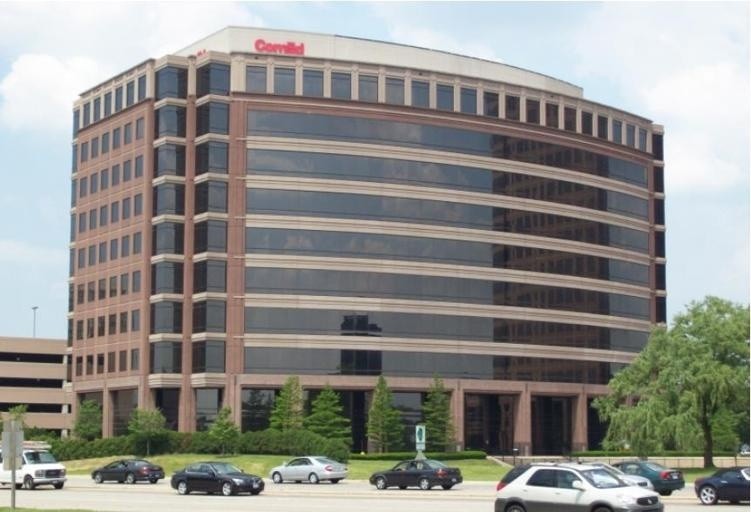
[0,438,74,490]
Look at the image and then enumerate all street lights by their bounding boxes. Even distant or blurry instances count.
[28,306,41,340]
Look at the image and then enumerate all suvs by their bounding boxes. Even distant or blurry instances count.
[493,461,665,512]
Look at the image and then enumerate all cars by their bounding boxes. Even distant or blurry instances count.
[366,459,464,490]
[169,458,268,497]
[90,457,166,488]
[270,453,351,485]
[577,458,751,503]
